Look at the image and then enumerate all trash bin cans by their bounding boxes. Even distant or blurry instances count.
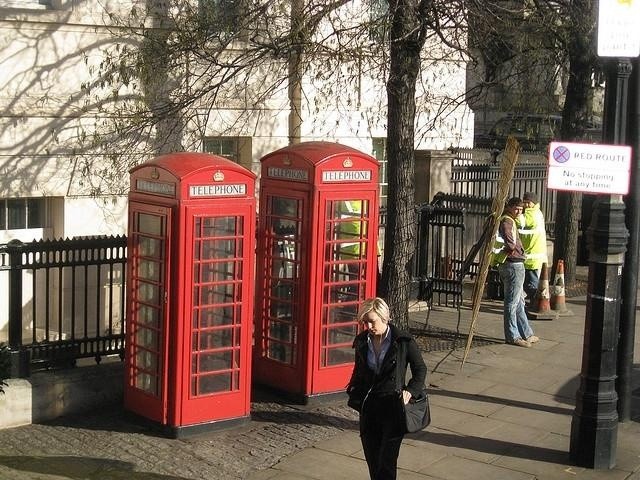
[487,270,504,300]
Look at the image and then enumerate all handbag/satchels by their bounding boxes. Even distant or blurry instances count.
[401,393,431,433]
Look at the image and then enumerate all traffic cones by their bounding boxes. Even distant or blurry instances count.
[549,259,576,318]
[524,261,559,321]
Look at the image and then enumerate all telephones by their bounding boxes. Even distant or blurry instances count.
[276,232,300,278]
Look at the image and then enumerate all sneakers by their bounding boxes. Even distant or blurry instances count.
[515,335,539,347]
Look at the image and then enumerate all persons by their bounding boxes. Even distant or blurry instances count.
[489,197,539,347]
[344,296,426,479]
[515,192,548,307]
[329,198,389,332]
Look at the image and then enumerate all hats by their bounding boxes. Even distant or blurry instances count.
[524,192,538,204]
